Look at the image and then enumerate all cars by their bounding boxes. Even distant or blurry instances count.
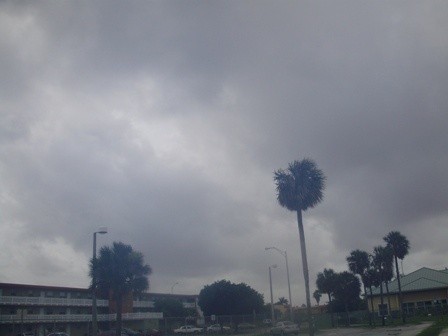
[205,322,232,334]
[269,320,299,336]
[15,327,157,336]
[235,321,254,333]
[174,325,204,335]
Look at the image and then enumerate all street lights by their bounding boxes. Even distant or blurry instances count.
[90,226,109,335]
[266,246,295,322]
[269,264,279,327]
